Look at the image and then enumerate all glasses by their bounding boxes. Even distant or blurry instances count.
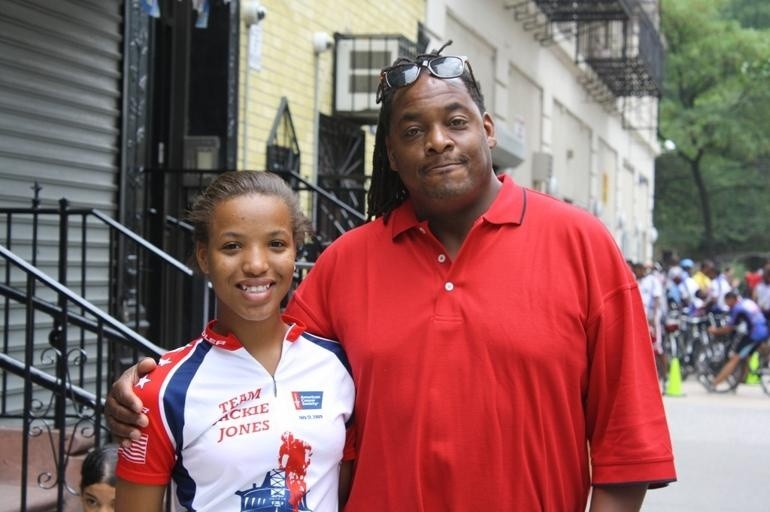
[375,56,482,104]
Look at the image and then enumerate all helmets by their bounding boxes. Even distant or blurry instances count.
[667,258,696,281]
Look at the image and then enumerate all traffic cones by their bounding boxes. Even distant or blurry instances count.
[665,358,684,397]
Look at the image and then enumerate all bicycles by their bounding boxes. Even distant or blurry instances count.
[654,307,770,393]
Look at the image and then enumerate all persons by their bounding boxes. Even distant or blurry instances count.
[111,169,357,512]
[626,251,770,393]
[81,447,118,512]
[102,53,678,511]
